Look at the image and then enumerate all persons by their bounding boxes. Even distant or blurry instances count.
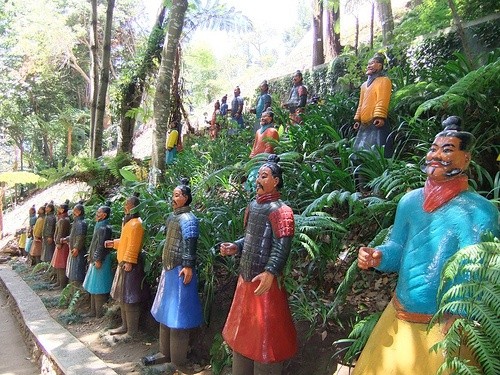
[348,52,392,193]
[41,200,55,270]
[220,154,299,375]
[219,94,228,117]
[284,69,308,125]
[208,93,223,141]
[144,178,199,373]
[45,198,71,289]
[231,85,245,129]
[352,116,500,375]
[165,118,179,166]
[80,201,112,321]
[250,80,272,134]
[61,201,87,293]
[19,200,47,265]
[104,191,144,342]
